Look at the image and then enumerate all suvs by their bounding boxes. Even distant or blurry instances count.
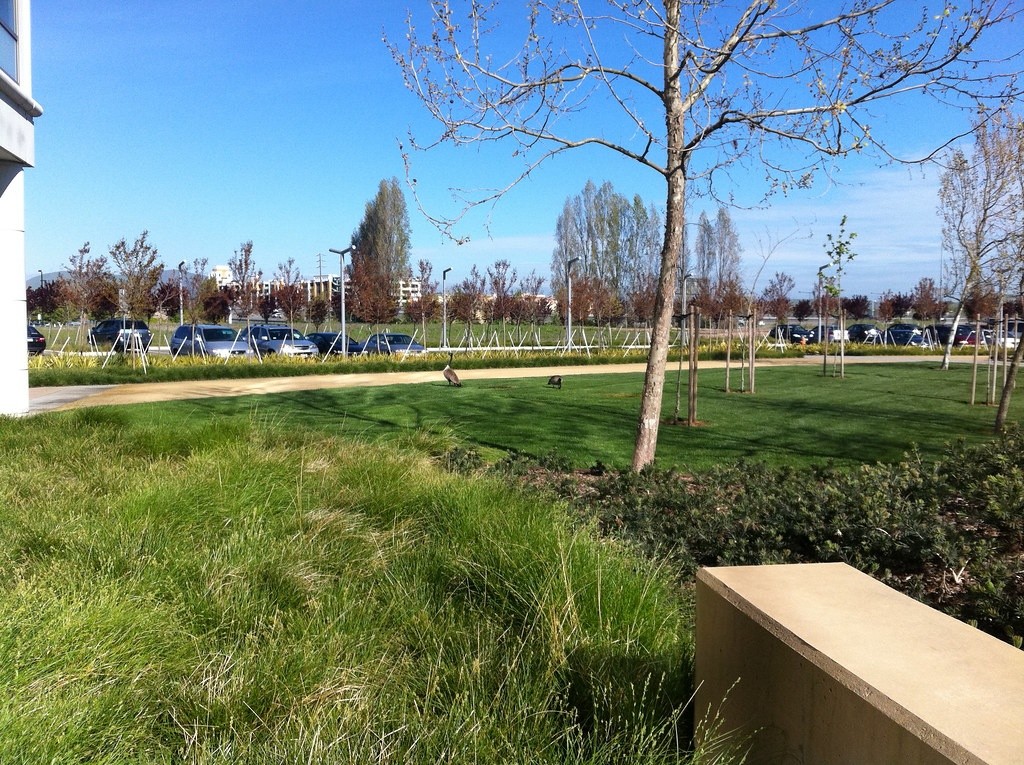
[87,317,153,355]
[240,322,319,358]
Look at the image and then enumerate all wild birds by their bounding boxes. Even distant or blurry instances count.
[548,375,563,389]
[442,353,461,387]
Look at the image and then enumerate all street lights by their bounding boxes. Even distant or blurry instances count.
[178,260,185,326]
[329,245,356,359]
[567,256,581,352]
[683,272,693,345]
[818,262,834,343]
[442,267,452,347]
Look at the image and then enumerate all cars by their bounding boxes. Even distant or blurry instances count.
[980,328,1021,349]
[304,332,370,359]
[985,319,1024,342]
[27,325,47,355]
[170,323,255,358]
[845,324,885,345]
[360,333,429,356]
[769,324,814,345]
[924,324,981,346]
[30,320,82,327]
[883,323,930,346]
[808,325,850,343]
